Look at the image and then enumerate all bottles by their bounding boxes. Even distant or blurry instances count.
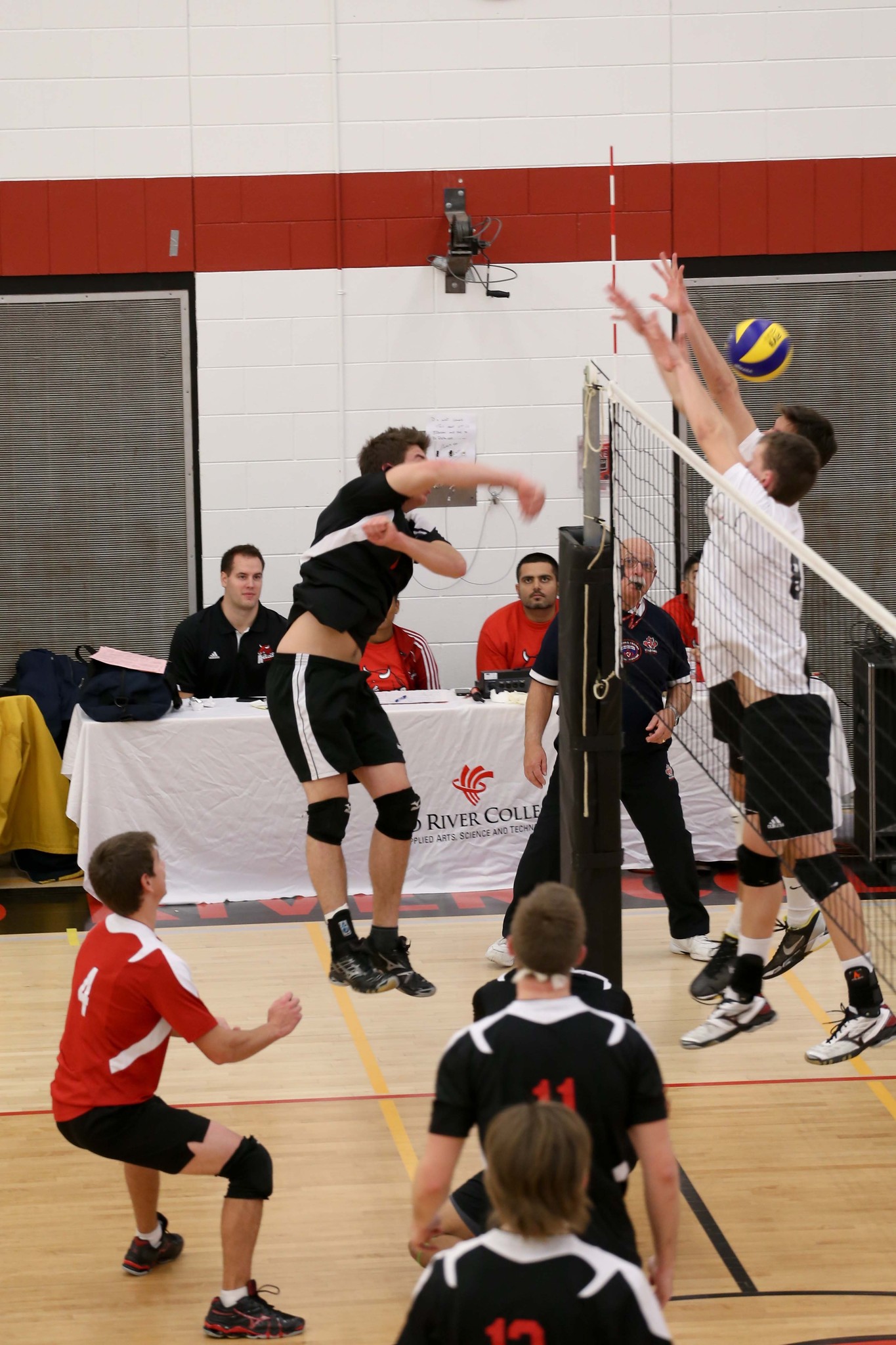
[685,647,696,695]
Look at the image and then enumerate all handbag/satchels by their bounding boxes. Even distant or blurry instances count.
[74,646,182,721]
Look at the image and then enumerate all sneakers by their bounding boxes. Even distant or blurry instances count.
[803,996,895,1065]
[680,985,778,1051]
[670,934,718,962]
[360,935,436,998]
[762,909,831,980]
[122,1211,184,1275]
[202,1279,308,1340]
[688,932,738,1005]
[485,936,515,967]
[328,938,400,994]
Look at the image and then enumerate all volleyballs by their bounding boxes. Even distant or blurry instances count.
[724,319,793,382]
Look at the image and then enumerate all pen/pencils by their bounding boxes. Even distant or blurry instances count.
[395,695,406,702]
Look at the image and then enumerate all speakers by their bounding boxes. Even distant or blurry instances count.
[853,647,896,862]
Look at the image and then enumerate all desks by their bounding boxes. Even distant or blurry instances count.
[0,695,80,855]
[62,677,854,905]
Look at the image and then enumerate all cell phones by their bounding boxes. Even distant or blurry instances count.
[454,688,471,695]
[236,695,266,703]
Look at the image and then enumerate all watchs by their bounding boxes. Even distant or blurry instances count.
[665,705,679,727]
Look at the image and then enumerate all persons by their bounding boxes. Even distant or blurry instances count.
[163,544,294,699]
[271,426,536,994]
[49,829,306,1340]
[358,250,896,1311]
[397,1103,672,1345]
